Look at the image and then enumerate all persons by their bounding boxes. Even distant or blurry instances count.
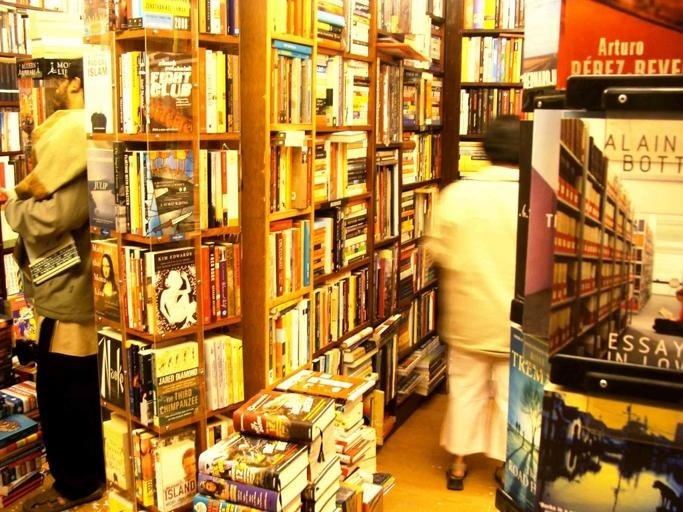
[428,114,523,494]
[3,63,106,511]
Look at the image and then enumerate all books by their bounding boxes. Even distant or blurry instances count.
[548,120,656,357]
[0,206,51,509]
[1,1,82,190]
[457,0,523,176]
[80,1,446,510]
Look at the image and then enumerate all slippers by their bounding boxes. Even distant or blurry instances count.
[446,463,468,489]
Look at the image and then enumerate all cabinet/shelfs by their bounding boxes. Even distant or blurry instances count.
[0,0,528,512]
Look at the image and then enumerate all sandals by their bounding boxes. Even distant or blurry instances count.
[23,486,102,512]
